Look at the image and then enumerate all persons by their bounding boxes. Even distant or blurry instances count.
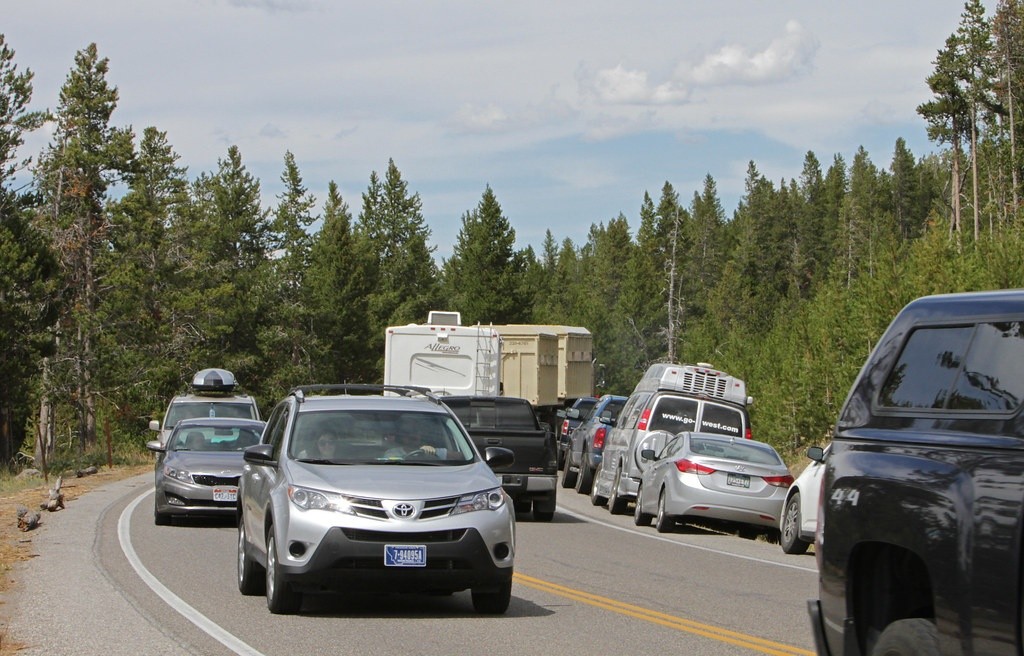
[237,428,256,451]
[383,416,465,461]
[305,425,343,460]
[185,432,208,451]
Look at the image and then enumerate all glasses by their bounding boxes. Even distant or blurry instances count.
[318,440,335,447]
[399,430,418,436]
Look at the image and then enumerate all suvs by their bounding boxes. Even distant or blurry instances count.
[236,382,518,614]
[156,365,260,482]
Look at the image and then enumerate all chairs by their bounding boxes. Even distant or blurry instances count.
[236,436,259,446]
[185,432,205,448]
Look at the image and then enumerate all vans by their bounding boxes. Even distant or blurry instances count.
[807,292,1024,656]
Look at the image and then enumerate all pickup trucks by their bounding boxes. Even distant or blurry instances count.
[442,397,559,519]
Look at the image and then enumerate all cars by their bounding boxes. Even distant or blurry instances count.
[145,416,269,526]
[555,363,833,553]
